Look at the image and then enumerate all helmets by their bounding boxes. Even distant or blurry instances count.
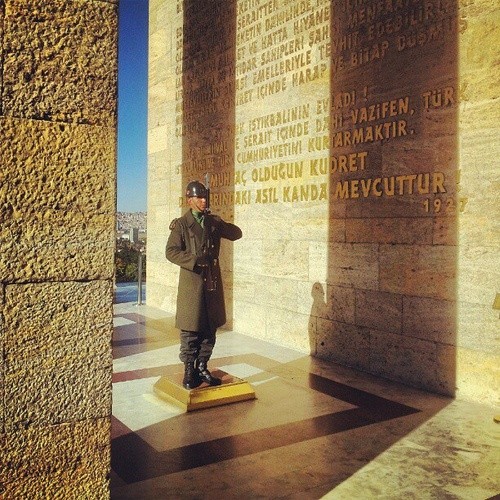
[185,179,208,198]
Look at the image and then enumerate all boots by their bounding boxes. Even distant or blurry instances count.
[182,360,199,389]
[195,359,222,386]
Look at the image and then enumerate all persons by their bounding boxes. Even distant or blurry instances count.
[164,181,243,391]
